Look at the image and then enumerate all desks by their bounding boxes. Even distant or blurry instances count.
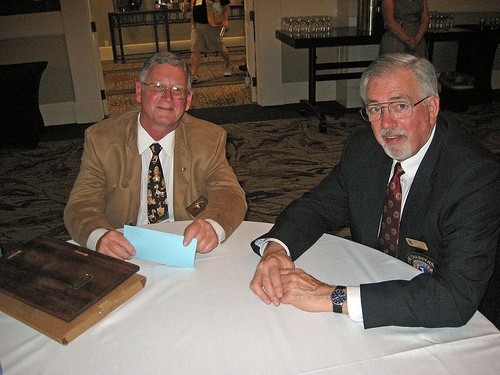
[107,3,244,66]
[0,219,500,375]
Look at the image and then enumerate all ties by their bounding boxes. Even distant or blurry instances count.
[147,143,169,224]
[376,162,405,257]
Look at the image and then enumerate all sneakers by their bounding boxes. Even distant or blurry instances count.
[222,64,232,77]
[190,75,201,83]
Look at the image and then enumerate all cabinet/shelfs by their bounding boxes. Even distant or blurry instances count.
[275,12,500,133]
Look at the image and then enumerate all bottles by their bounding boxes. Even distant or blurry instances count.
[281,15,331,32]
[428,12,455,29]
[480,13,500,31]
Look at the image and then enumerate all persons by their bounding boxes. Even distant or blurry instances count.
[63,49,248,261]
[249,53,500,334]
[379,0,430,59]
[190,0,236,84]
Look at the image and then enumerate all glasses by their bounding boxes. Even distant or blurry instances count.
[140,82,190,99]
[361,96,432,121]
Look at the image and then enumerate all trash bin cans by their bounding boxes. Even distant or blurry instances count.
[438,71,474,112]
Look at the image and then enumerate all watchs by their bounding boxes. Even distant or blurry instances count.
[331,285,347,313]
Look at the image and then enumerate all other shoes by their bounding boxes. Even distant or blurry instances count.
[239,65,247,71]
